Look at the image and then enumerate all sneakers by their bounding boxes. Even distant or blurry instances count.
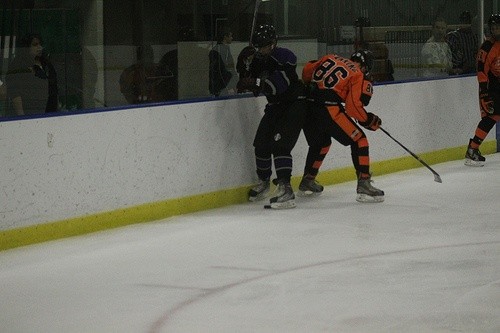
[269,179,297,208]
[299,176,324,195]
[356,179,384,203]
[464,138,486,167]
[247,175,271,201]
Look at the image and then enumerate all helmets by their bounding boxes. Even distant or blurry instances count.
[350,50,373,70]
[252,24,279,47]
[488,14,500,40]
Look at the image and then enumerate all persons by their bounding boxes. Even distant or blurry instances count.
[121,46,162,103]
[464,15,500,166]
[7,37,51,117]
[448,9,484,73]
[49,23,97,111]
[160,23,232,98]
[237,26,298,210]
[419,18,455,77]
[350,17,395,83]
[235,46,257,94]
[209,30,240,96]
[303,50,385,203]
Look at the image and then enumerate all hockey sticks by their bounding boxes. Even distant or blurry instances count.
[378,125,443,183]
[244,0,261,72]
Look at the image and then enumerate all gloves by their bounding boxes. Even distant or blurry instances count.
[358,112,382,131]
[479,94,495,115]
[236,76,261,97]
